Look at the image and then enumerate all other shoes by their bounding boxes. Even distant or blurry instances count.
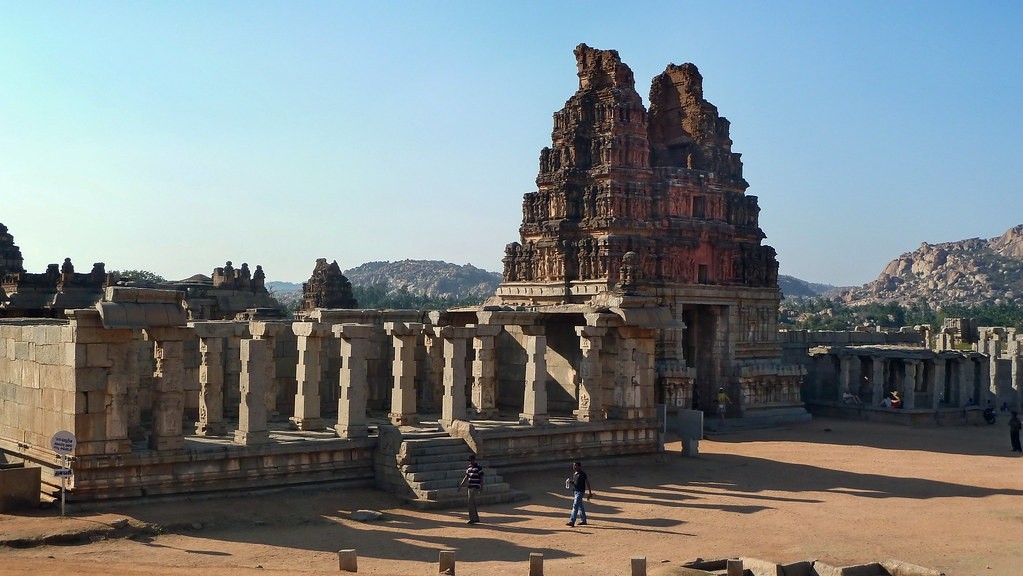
[467,519,480,525]
[565,522,574,527]
[577,521,587,525]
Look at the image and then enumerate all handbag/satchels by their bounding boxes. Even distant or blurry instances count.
[719,404,723,408]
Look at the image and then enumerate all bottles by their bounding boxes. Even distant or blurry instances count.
[566,478,570,489]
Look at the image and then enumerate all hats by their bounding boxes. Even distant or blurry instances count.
[720,388,723,390]
[467,454,476,462]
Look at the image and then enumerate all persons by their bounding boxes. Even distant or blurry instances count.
[565,460,592,527]
[1000,402,1010,412]
[879,391,903,408]
[842,390,863,405]
[457,455,484,525]
[985,399,993,408]
[966,398,975,408]
[1008,410,1023,453]
[715,387,733,423]
[939,392,944,402]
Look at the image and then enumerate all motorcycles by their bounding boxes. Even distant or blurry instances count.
[984,407,998,425]
